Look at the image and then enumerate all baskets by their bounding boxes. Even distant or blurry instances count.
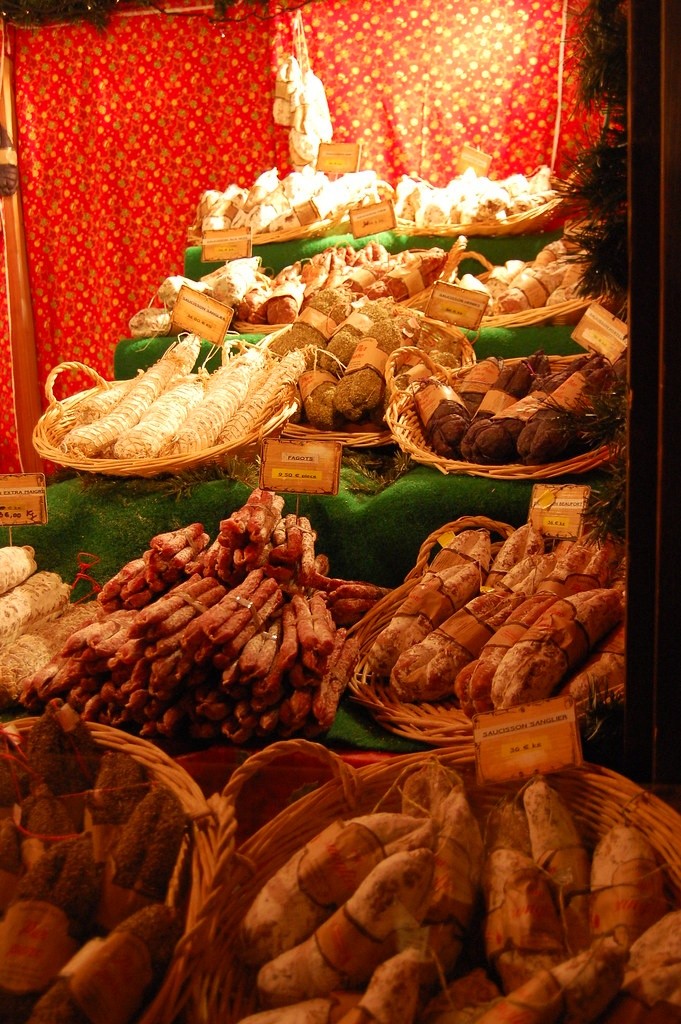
[392,173,579,237]
[0,717,216,1024]
[430,251,621,331]
[32,340,301,480]
[231,234,467,333]
[250,302,471,448]
[185,180,395,245]
[383,345,627,481]
[189,737,681,1024]
[344,514,628,748]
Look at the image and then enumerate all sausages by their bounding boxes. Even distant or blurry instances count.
[0,56,681,1024]
